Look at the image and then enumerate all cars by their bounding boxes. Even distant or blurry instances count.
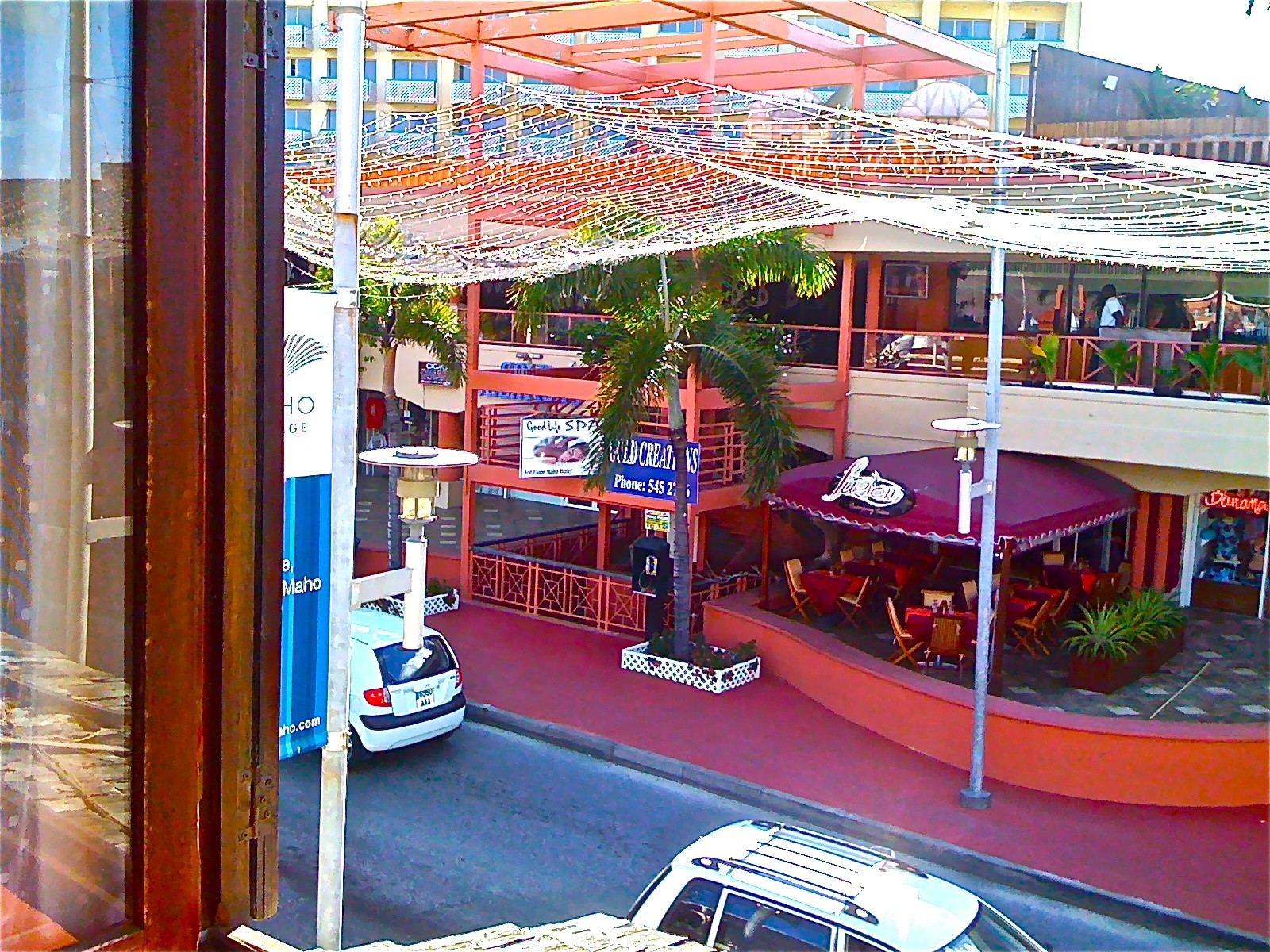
[347,607,468,764]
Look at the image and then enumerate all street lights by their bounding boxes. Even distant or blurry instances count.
[936,413,1003,811]
[316,442,479,952]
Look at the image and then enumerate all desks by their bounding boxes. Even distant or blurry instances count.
[903,601,979,675]
[886,549,935,579]
[798,569,864,626]
[1008,581,1061,605]
[991,593,1036,633]
[844,556,910,595]
[1057,564,1107,592]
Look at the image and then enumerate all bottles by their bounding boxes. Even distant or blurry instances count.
[932,599,937,612]
[949,601,954,615]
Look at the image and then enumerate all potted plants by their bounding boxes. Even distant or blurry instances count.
[1147,359,1185,398]
[1055,582,1189,695]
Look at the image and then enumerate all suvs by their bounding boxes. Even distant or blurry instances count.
[621,818,1054,951]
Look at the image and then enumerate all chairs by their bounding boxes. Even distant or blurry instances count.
[781,541,1124,685]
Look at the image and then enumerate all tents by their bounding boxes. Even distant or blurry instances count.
[759,447,1142,698]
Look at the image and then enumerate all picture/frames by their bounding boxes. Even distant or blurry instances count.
[884,263,929,298]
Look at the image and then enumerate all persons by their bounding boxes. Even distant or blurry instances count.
[1097,284,1128,327]
[535,435,588,465]
